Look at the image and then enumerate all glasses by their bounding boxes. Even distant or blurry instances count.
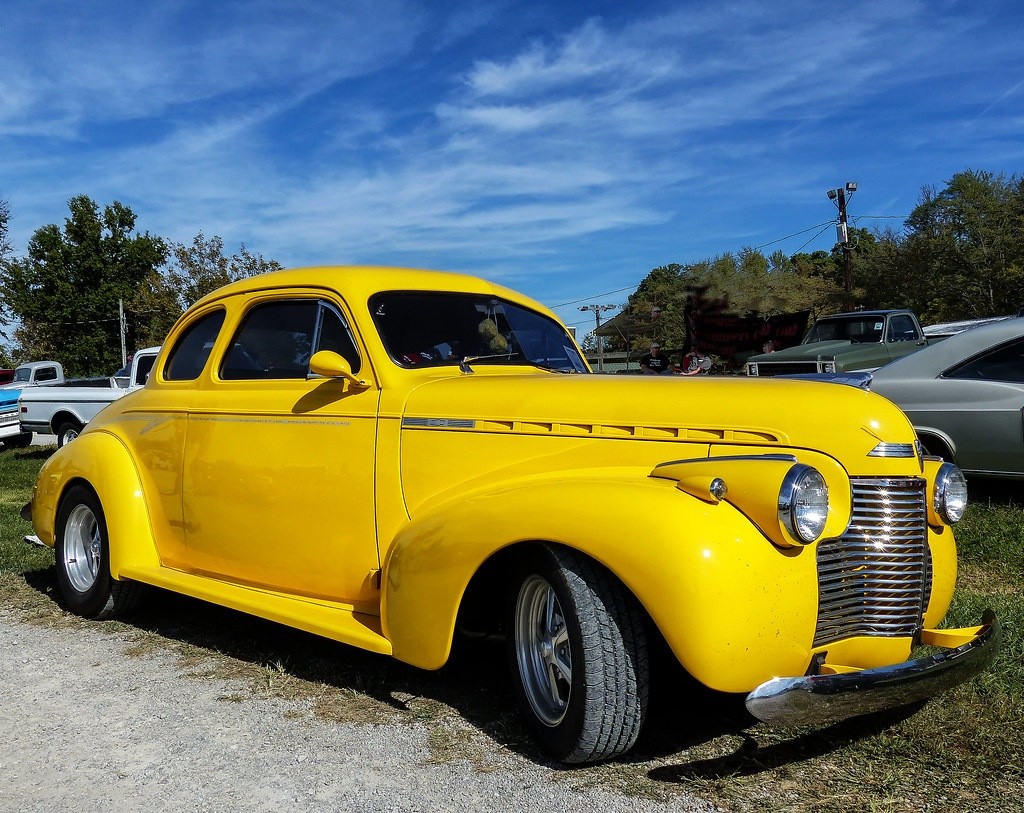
[652,347,661,349]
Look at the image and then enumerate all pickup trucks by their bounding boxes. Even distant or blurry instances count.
[744,308,1012,377]
[17,347,162,450]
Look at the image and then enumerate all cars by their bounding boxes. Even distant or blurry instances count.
[14,265,1002,768]
[771,317,1024,482]
[0,360,65,448]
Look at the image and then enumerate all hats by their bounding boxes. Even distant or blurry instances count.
[651,343,659,348]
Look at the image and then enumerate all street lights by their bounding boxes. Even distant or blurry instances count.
[577,304,618,371]
[826,181,858,312]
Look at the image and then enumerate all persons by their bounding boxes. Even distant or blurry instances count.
[638,334,711,377]
[767,342,775,353]
